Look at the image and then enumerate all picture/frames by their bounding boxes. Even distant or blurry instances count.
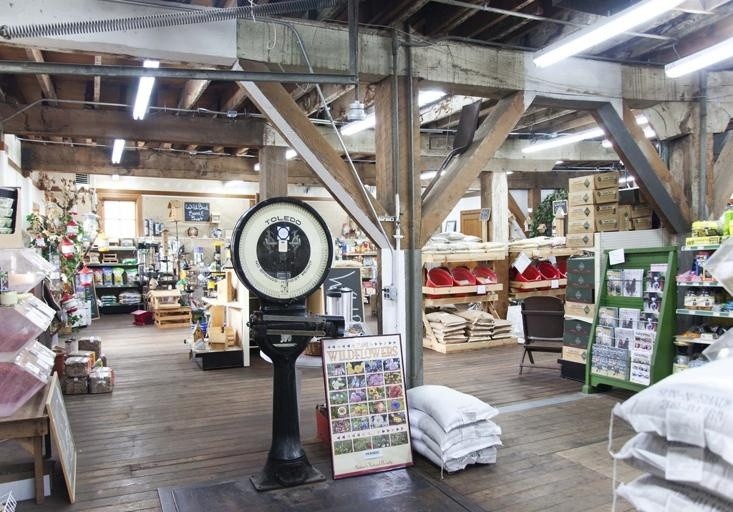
[321,334,415,481]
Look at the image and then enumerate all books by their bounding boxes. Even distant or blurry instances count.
[595,324,656,351]
[591,343,652,387]
[599,291,662,331]
[605,264,668,298]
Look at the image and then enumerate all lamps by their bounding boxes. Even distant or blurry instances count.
[132,58,160,121]
[520,123,606,158]
[663,36,733,78]
[111,138,126,165]
[533,1,685,71]
[339,89,451,137]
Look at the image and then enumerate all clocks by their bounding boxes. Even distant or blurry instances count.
[228,195,334,306]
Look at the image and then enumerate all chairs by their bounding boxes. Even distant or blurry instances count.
[519,293,563,381]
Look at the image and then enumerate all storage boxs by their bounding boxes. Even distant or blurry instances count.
[60,336,114,397]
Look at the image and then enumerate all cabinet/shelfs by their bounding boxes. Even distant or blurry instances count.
[512,240,584,301]
[1,376,58,505]
[672,243,733,377]
[581,245,678,399]
[335,238,381,307]
[84,238,143,315]
[184,272,251,371]
[420,242,519,353]
[557,256,597,383]
